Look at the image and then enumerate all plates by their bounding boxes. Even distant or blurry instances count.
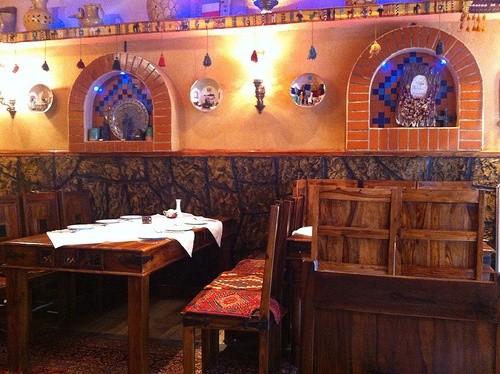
[95,219,124,224]
[137,233,170,240]
[67,224,95,230]
[165,227,193,231]
[185,222,207,225]
[120,215,142,219]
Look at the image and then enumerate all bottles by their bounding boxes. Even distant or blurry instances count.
[175,199,183,217]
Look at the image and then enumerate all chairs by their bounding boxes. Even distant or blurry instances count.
[181,195,305,374]
[0,190,106,338]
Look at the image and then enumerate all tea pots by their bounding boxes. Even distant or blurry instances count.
[163,208,178,219]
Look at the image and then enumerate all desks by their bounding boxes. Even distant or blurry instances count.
[0,216,235,374]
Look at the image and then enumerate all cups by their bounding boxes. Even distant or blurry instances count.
[142,216,152,224]
